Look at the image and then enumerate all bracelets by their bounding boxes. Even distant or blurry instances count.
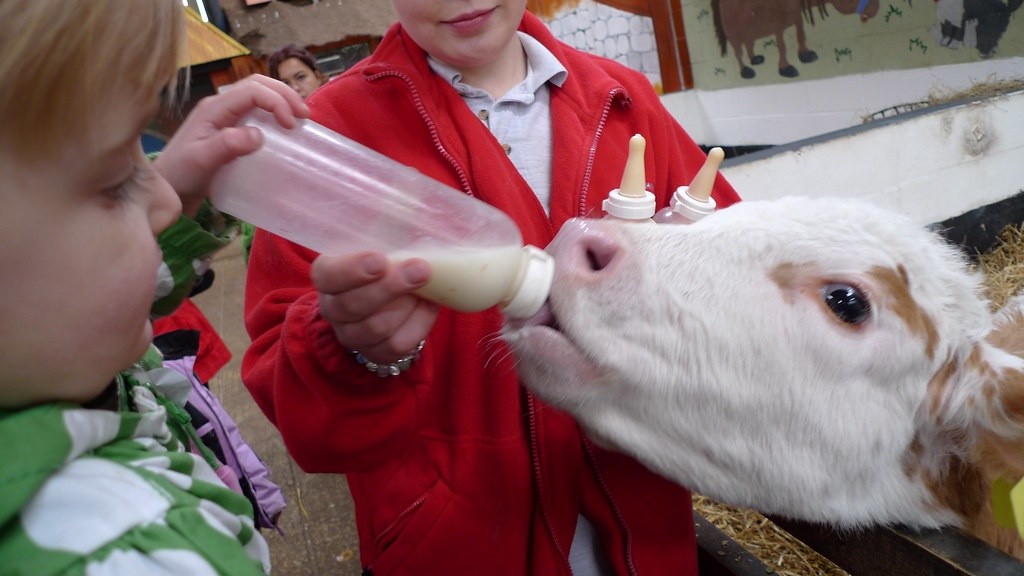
[350,338,425,381]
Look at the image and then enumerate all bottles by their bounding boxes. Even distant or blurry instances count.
[210,104,554,317]
[652,148,725,229]
[597,133,658,226]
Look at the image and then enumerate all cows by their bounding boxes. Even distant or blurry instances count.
[478,190,1024,563]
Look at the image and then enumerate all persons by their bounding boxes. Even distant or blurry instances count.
[268,44,327,106]
[242,0,742,576]
[0,2,309,576]
